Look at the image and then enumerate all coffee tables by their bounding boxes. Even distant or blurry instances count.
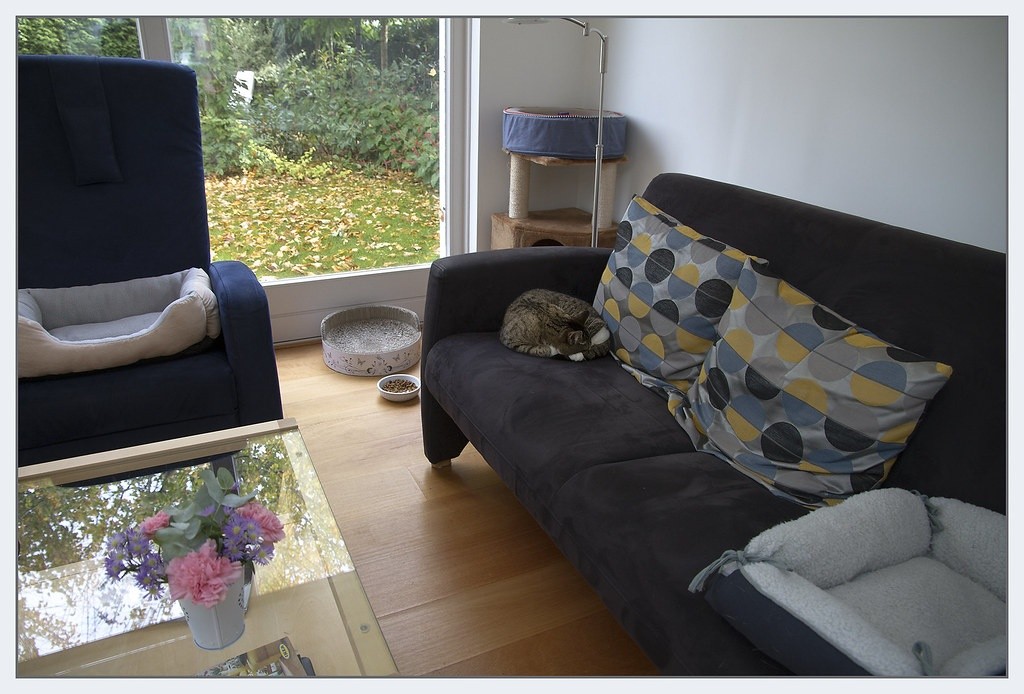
[16,425,403,678]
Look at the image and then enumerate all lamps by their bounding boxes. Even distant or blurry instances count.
[507,16,610,249]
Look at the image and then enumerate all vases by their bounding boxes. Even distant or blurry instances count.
[178,566,247,650]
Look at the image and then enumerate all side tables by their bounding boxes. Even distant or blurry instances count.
[490,206,621,250]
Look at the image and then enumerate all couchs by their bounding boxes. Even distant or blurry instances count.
[416,173,1008,678]
[17,54,284,467]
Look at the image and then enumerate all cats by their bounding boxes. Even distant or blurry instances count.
[499,288,611,361]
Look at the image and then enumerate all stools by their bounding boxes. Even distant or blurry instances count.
[503,105,627,227]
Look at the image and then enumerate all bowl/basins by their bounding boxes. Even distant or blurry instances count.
[377,374,420,402]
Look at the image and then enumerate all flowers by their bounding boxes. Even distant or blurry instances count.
[103,468,286,609]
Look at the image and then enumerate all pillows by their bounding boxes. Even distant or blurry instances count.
[672,256,955,512]
[591,188,769,394]
